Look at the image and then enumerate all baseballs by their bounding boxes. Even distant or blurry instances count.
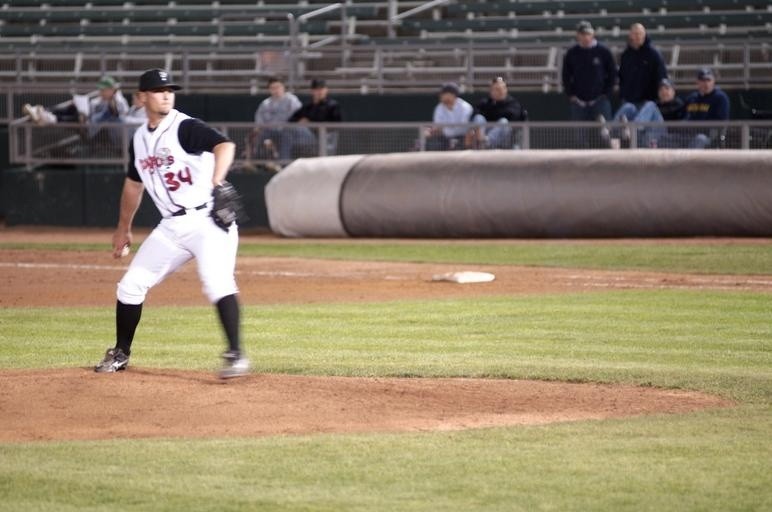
[122,243,131,256]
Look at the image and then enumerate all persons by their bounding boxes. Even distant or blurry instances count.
[243,78,343,173]
[118,91,150,126]
[254,74,302,130]
[424,81,474,150]
[465,76,527,149]
[23,74,130,146]
[93,68,251,379]
[596,77,684,148]
[668,68,730,148]
[562,21,615,147]
[618,22,668,111]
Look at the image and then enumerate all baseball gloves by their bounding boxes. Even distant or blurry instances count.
[210,182,250,232]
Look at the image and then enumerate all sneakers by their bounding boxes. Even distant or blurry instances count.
[599,114,609,139]
[23,103,56,125]
[619,113,630,140]
[241,138,283,172]
[219,351,250,379]
[94,348,129,371]
[463,129,488,151]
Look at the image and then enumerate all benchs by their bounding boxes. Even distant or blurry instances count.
[0,0,772,162]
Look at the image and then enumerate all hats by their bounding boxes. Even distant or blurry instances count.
[138,68,183,90]
[310,78,326,89]
[438,82,457,94]
[96,75,115,90]
[577,22,594,35]
[699,67,714,80]
[658,78,673,86]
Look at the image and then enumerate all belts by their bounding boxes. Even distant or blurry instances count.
[174,203,206,216]
